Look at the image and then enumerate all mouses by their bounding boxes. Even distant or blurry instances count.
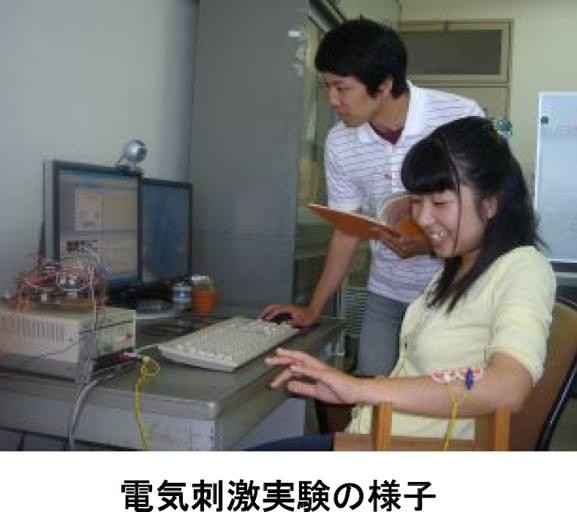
[262,313,293,325]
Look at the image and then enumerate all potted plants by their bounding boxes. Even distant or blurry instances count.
[190,274,216,316]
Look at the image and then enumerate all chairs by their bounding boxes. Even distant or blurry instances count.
[313,296,577,451]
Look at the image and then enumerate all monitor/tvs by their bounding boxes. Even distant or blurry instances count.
[43,159,142,309]
[142,178,193,313]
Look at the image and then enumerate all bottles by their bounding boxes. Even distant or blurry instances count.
[172,275,193,309]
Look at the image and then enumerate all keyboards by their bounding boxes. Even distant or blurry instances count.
[158,315,300,373]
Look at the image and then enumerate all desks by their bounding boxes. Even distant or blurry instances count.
[0,306,349,451]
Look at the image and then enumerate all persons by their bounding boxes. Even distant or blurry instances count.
[243,117,556,451]
[255,21,486,377]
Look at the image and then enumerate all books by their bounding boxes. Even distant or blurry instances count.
[308,191,434,254]
